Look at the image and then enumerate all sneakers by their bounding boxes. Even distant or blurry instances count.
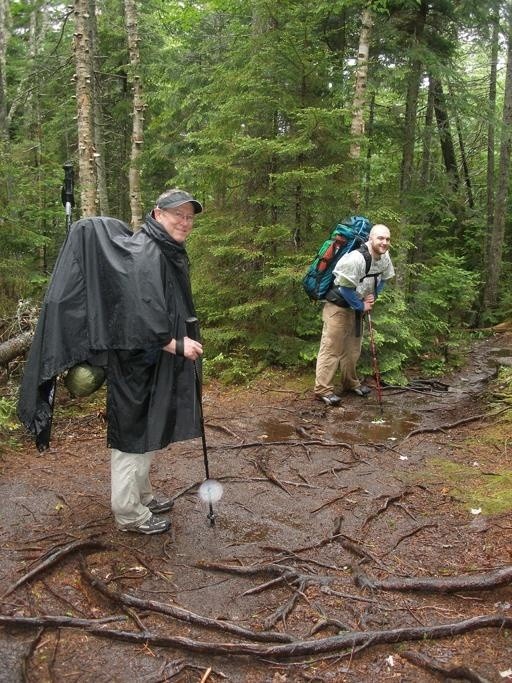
[147,498,175,513]
[118,515,172,534]
[315,392,341,405]
[352,386,372,395]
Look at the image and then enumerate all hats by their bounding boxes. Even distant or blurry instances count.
[157,189,203,213]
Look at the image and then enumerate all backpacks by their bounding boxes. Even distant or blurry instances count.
[302,216,373,302]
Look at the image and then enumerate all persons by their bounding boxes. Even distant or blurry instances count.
[310,222,397,405]
[102,188,205,534]
